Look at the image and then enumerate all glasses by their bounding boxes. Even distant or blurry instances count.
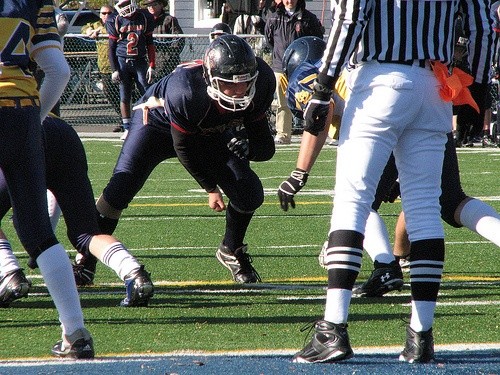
[146,3,158,6]
[100,11,113,16]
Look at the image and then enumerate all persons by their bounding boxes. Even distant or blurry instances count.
[1,113,157,307]
[55,0,499,154]
[65,29,280,285]
[277,36,500,302]
[1,0,99,361]
[289,0,497,365]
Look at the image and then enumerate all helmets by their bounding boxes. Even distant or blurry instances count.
[202,35,259,111]
[114,0,138,17]
[281,36,327,84]
[210,22,231,42]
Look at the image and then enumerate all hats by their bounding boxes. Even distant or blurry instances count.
[143,0,168,6]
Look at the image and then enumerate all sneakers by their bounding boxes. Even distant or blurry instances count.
[51,327,95,358]
[69,264,95,287]
[215,239,258,283]
[119,264,155,307]
[0,268,30,304]
[351,260,404,299]
[398,325,435,365]
[288,318,355,365]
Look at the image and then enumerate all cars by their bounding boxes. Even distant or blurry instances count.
[61,10,102,34]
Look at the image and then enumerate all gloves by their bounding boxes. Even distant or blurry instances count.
[455,82,488,145]
[302,91,330,136]
[111,70,121,86]
[277,168,309,213]
[146,66,154,84]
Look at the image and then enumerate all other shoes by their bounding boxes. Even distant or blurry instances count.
[453,135,500,147]
